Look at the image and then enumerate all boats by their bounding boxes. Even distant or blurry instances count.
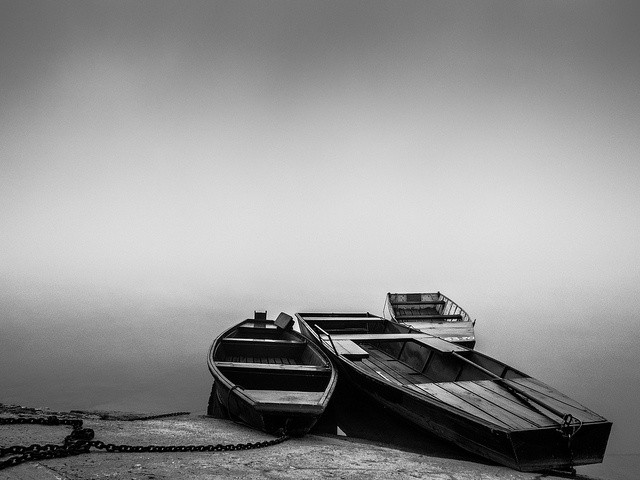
[208,309,338,431]
[388,290,475,344]
[296,313,612,472]
